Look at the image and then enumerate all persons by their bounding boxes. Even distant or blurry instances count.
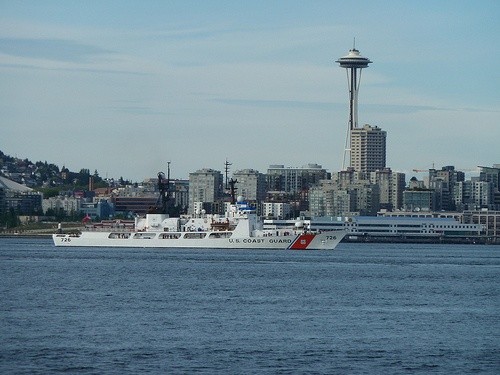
[58,221,62,234]
[304,225,308,235]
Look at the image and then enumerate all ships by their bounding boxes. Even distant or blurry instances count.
[52,158,350,248]
